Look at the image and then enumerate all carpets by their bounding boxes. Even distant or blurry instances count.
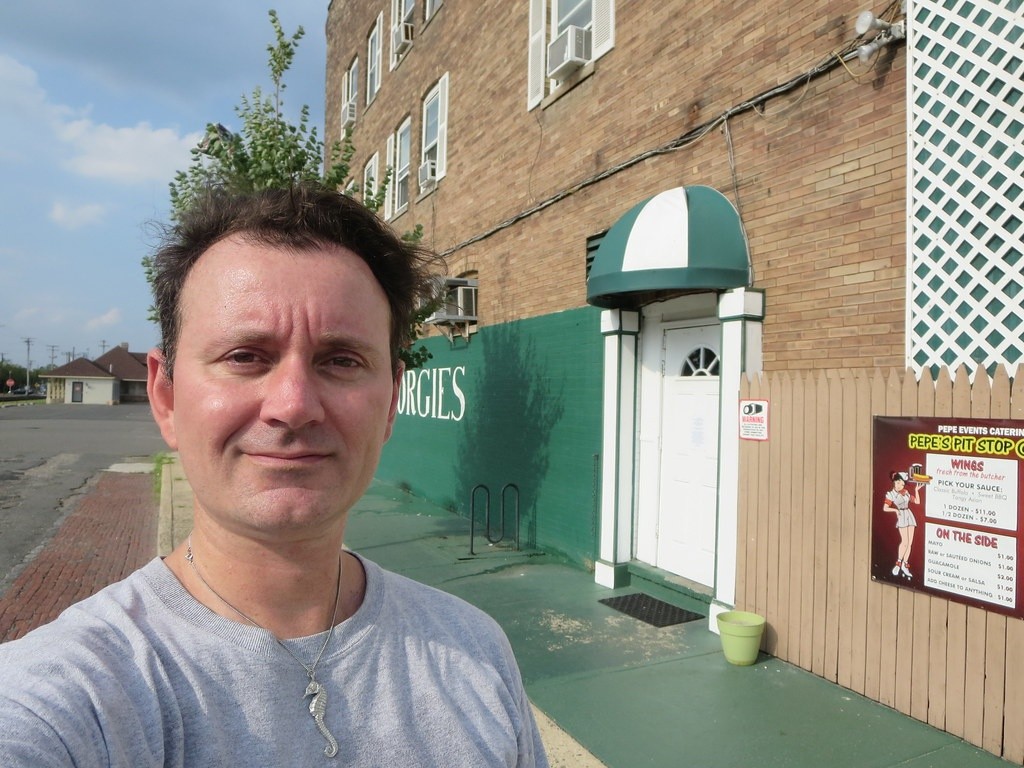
[598,592,705,628]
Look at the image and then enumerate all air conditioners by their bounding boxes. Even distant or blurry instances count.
[418,160,435,187]
[340,103,356,128]
[547,25,591,79]
[393,23,414,54]
[418,277,476,325]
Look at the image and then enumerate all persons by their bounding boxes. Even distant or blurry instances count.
[0,176,552,768]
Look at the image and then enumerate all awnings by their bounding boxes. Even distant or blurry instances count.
[585,185,749,311]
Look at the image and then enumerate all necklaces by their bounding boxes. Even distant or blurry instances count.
[187,530,343,757]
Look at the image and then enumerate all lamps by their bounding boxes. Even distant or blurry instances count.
[855,11,904,63]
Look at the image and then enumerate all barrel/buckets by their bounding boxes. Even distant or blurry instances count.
[716,611,766,666]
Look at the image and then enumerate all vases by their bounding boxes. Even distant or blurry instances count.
[717,611,764,666]
